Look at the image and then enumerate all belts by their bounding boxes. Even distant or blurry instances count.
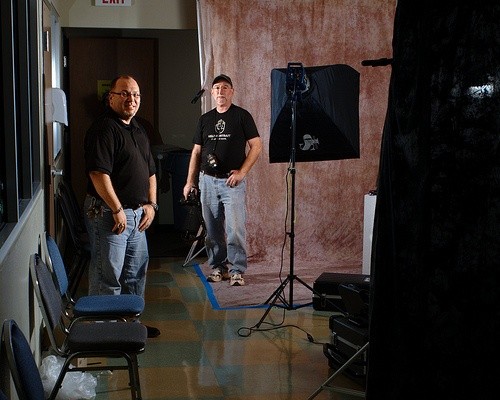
[199,168,234,179]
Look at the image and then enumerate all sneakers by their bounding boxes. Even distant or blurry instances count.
[230,273,245,286]
[207,269,228,282]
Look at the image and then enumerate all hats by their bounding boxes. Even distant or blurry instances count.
[211,74,232,85]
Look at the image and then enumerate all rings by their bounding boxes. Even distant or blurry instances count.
[233,182,235,184]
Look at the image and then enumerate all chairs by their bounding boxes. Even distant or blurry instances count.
[56,183,91,298]
[4,319,44,400]
[29,231,147,400]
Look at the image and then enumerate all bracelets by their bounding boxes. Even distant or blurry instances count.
[148,200,159,211]
[112,206,123,214]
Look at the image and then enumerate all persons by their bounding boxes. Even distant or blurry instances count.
[84,74,161,354]
[183,74,262,287]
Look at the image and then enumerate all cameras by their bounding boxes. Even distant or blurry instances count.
[179,188,201,207]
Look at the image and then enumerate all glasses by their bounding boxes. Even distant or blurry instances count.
[211,86,233,91]
[110,91,142,98]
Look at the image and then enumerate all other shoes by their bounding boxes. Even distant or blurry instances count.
[139,322,160,338]
